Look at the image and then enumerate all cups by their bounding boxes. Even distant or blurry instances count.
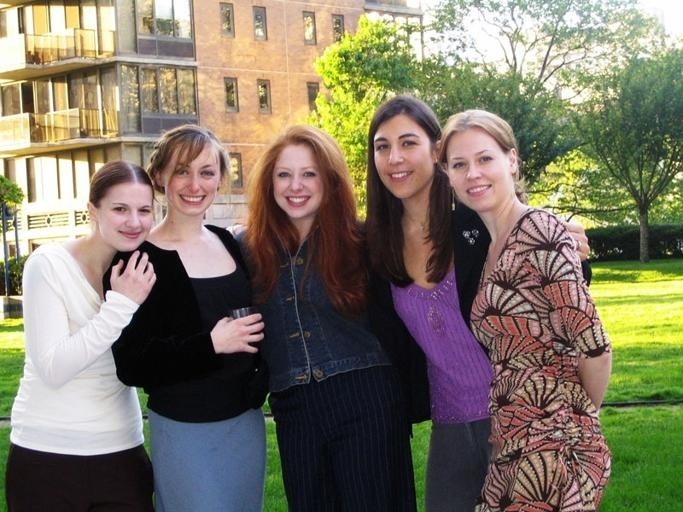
[232,306,259,346]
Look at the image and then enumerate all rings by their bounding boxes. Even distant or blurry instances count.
[577,240,582,252]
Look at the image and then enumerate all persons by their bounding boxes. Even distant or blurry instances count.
[0,159,158,511]
[357,95,594,511]
[104,121,279,511]
[228,123,422,511]
[432,106,617,511]
